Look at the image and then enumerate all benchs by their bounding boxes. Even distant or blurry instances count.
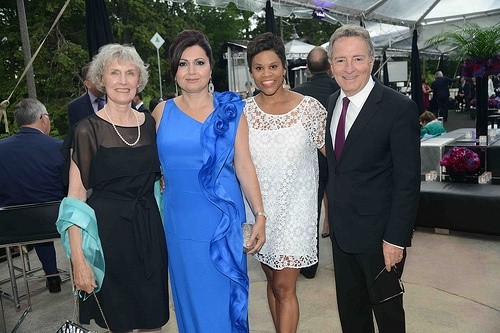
[416,181,500,237]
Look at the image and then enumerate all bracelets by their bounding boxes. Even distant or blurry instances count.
[255,212,266,219]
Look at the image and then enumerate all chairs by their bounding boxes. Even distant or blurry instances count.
[0,202,77,333]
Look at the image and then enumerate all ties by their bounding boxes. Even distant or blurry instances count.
[96,99,104,111]
[335,97,350,162]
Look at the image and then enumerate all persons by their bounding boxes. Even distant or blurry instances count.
[241,33,328,333]
[65,48,476,278]
[325,25,420,333]
[0,98,64,293]
[151,29,266,333]
[66,42,170,333]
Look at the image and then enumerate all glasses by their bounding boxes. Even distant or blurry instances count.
[40,114,52,121]
[371,264,405,305]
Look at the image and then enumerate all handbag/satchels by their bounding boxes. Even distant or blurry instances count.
[240,223,257,253]
[55,288,113,333]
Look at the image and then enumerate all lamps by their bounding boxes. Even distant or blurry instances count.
[360,16,366,28]
[312,8,325,20]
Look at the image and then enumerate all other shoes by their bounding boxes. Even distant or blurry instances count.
[46,276,61,293]
[299,268,313,278]
[321,230,330,237]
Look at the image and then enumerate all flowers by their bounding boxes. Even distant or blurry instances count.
[488,99,500,108]
[439,147,479,171]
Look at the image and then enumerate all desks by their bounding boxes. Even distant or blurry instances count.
[420,128,500,175]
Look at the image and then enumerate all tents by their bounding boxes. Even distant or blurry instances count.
[286,39,317,60]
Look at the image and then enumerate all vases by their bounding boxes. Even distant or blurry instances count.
[456,171,467,183]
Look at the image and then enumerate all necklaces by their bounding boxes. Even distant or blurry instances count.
[103,104,140,146]
